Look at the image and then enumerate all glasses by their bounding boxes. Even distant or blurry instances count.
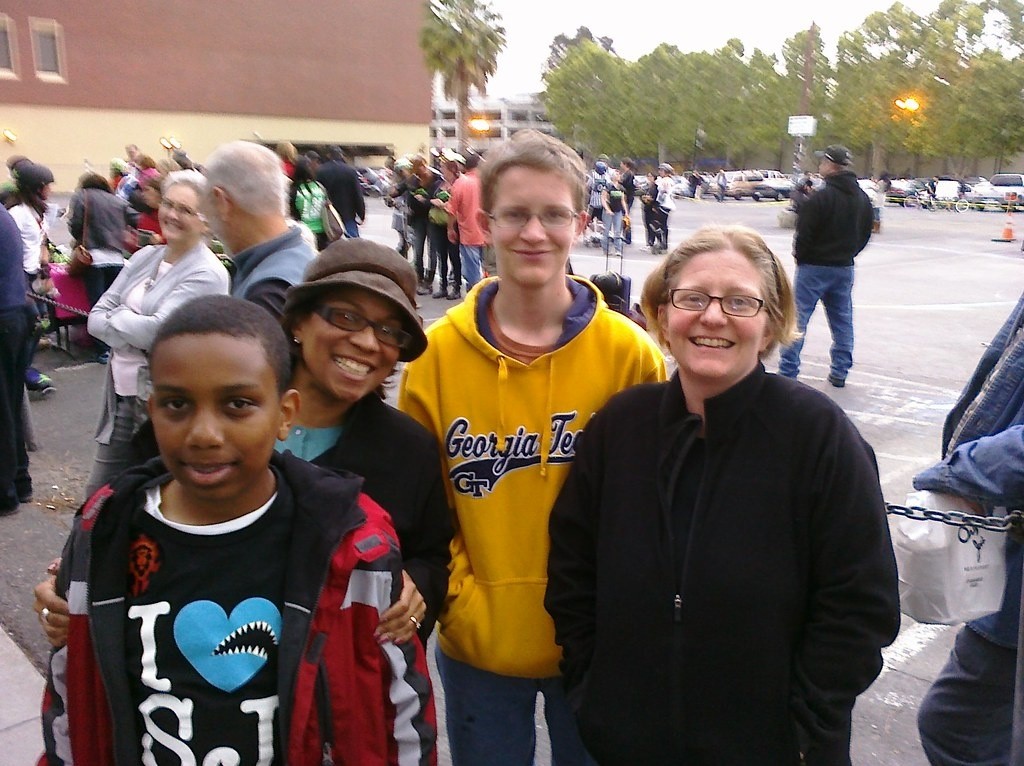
[319,305,412,351]
[162,199,198,217]
[489,209,581,229]
[670,288,765,319]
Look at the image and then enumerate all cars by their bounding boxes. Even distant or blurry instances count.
[632,169,826,202]
[886,180,914,207]
[932,180,961,202]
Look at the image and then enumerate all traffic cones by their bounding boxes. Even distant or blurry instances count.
[1000,210,1017,242]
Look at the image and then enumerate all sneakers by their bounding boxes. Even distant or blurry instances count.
[447,289,461,300]
[417,281,432,296]
[433,288,446,299]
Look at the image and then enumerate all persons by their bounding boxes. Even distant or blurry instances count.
[544,225,901,766]
[926,176,938,194]
[0,129,674,766]
[779,144,872,386]
[789,171,826,215]
[688,168,727,202]
[863,174,885,232]
[910,289,1024,766]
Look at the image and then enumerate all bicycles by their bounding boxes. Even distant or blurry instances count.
[903,188,970,213]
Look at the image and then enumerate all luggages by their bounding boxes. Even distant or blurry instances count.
[588,235,631,317]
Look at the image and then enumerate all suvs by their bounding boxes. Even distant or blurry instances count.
[989,173,1024,212]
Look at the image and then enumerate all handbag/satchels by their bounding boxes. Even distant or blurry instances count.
[322,202,345,243]
[65,246,93,279]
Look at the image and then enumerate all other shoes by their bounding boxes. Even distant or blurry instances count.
[827,375,845,388]
[23,367,53,391]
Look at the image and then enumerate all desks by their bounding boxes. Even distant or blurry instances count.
[50,264,89,349]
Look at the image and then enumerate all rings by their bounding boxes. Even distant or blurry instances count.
[41,608,49,624]
[410,617,421,629]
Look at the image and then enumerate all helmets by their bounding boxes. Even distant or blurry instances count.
[17,164,54,195]
[595,162,607,175]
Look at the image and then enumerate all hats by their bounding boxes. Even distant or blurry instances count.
[658,163,673,173]
[282,238,428,362]
[599,154,609,160]
[814,144,853,166]
